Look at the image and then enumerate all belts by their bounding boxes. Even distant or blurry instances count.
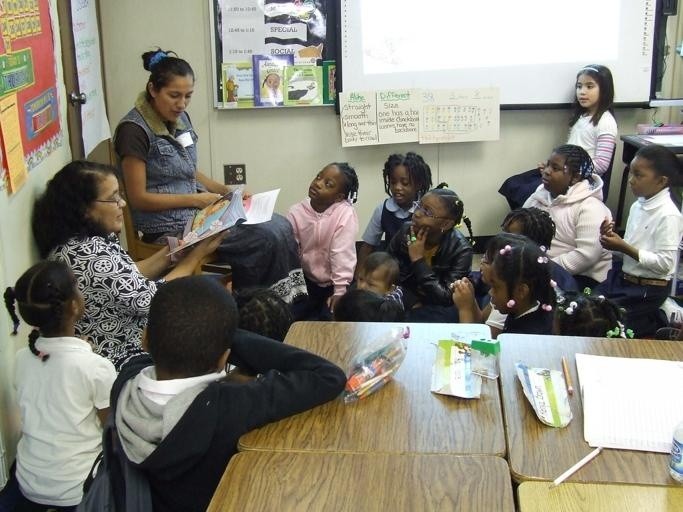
[623,273,669,286]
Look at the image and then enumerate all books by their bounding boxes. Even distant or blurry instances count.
[221,54,336,107]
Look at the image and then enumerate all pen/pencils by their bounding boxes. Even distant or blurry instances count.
[562,357,574,395]
[343,345,407,405]
[549,447,603,489]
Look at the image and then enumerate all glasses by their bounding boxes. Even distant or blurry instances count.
[412,200,450,221]
[95,190,127,207]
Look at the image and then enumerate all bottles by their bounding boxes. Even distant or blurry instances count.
[668,422,683,484]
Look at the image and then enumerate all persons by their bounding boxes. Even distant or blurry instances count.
[497,64,617,214]
[598,145,683,340]
[112,48,306,340]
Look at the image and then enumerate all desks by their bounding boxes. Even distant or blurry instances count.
[516,479,681,512]
[614,134,683,233]
[495,333,682,486]
[237,320,507,458]
[207,450,516,511]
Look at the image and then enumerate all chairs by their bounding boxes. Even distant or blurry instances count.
[107,139,232,289]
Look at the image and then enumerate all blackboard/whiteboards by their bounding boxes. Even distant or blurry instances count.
[209,0,343,110]
